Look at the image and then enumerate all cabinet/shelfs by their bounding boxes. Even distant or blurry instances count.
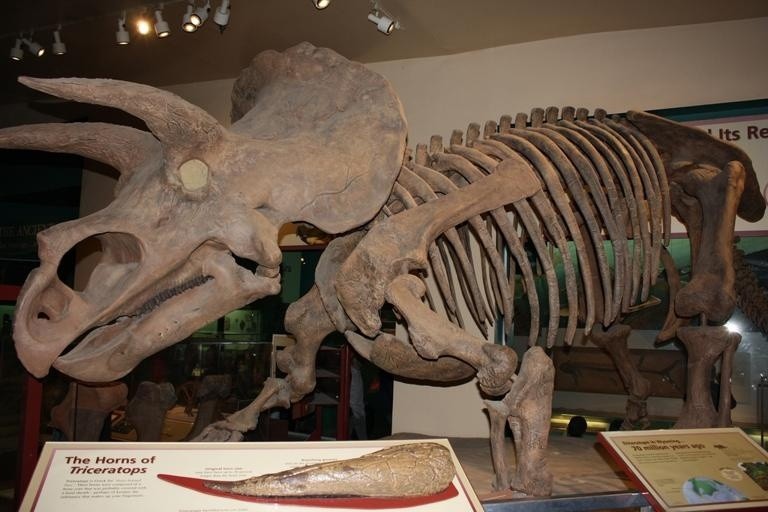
[300,344,350,441]
